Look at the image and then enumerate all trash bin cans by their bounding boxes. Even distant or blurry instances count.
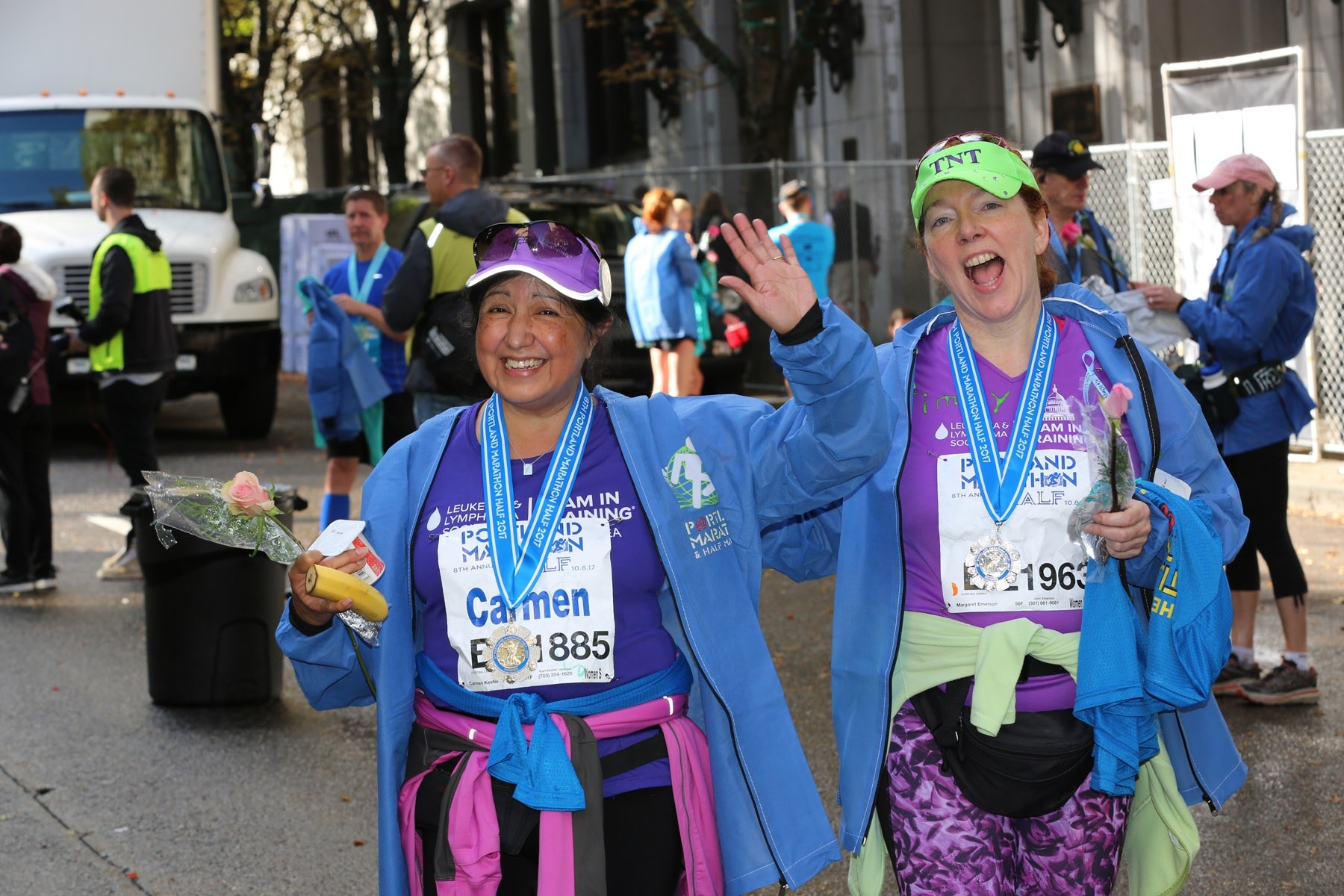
[123,488,298,710]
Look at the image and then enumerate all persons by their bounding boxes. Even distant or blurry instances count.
[66,165,179,584]
[763,174,837,300]
[268,220,896,896]
[1125,152,1324,706]
[622,178,748,396]
[0,221,64,592]
[1027,132,1129,298]
[295,133,530,536]
[755,130,1254,895]
[827,184,880,331]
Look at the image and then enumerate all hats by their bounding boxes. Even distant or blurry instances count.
[1192,153,1279,192]
[911,141,1042,234]
[465,226,612,307]
[1030,133,1106,180]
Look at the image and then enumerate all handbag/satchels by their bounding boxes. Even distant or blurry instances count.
[910,682,1098,817]
[1172,356,1287,419]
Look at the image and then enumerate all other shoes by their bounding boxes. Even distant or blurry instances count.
[96,531,149,581]
[0,567,60,593]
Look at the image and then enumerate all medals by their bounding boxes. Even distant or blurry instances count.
[482,617,542,686]
[962,533,1021,596]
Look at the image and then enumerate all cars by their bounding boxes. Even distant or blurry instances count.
[378,178,754,414]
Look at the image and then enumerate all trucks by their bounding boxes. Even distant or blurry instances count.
[0,0,284,441]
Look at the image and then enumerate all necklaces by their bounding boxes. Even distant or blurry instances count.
[502,435,558,477]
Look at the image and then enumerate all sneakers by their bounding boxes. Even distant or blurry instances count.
[1240,656,1321,707]
[1212,652,1260,695]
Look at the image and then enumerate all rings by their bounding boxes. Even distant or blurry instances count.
[773,255,782,260]
[1147,297,1154,305]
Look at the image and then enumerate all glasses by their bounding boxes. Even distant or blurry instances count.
[915,131,1011,172]
[470,220,601,269]
[417,167,445,177]
[345,184,379,197]
